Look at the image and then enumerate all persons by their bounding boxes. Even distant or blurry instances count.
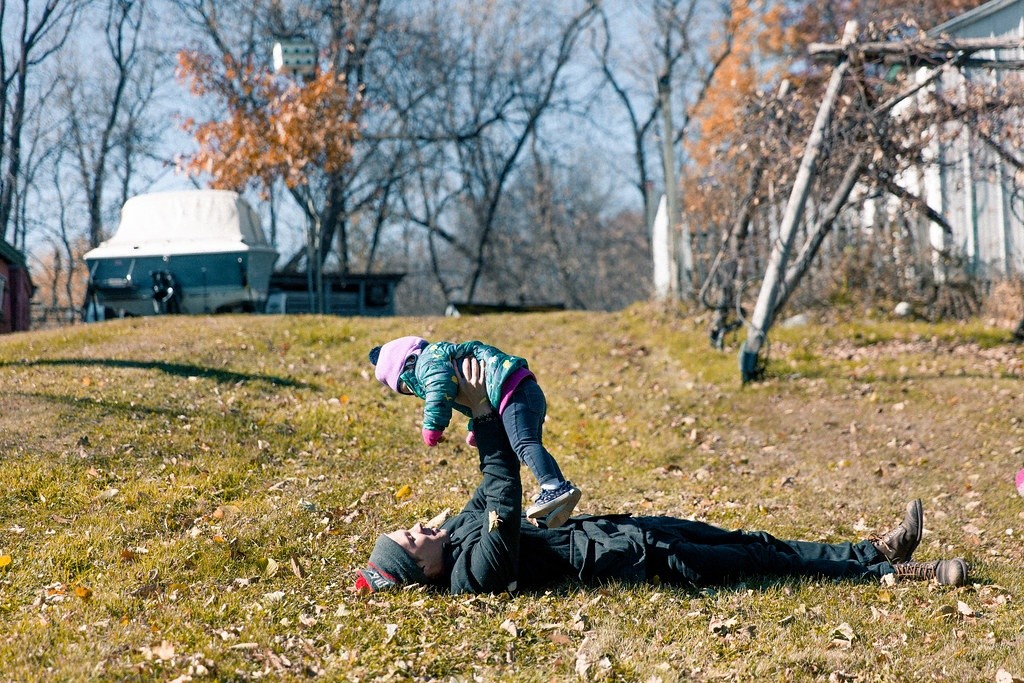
[369,336,582,529]
[356,357,967,595]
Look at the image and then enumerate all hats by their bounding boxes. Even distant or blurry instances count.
[352,533,446,591]
[369,334,424,395]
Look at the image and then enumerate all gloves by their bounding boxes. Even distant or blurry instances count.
[466,432,479,446]
[421,427,443,446]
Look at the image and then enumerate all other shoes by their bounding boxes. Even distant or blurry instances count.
[544,487,582,529]
[528,479,576,518]
[892,557,969,588]
[871,498,925,561]
